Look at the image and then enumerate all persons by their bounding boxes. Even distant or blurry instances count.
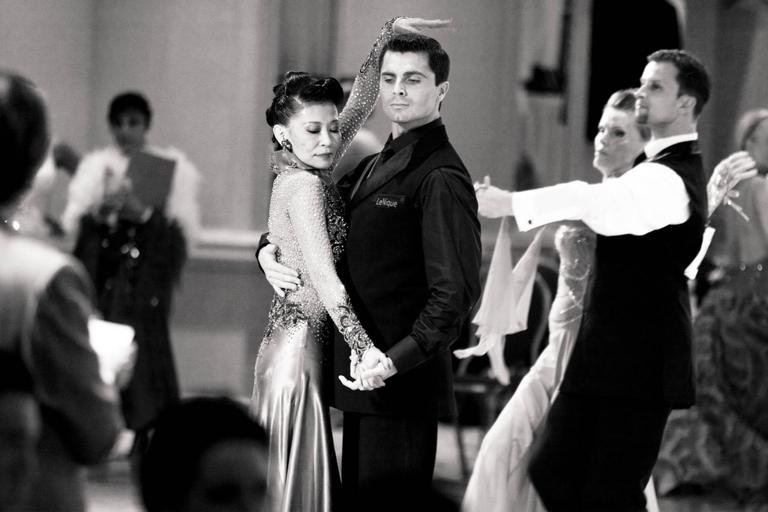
[60,92,200,453]
[10,136,83,243]
[468,86,757,512]
[477,48,708,512]
[256,31,485,512]
[243,16,461,512]
[127,393,278,512]
[0,65,118,512]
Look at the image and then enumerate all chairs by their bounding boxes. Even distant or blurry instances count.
[440,266,559,478]
